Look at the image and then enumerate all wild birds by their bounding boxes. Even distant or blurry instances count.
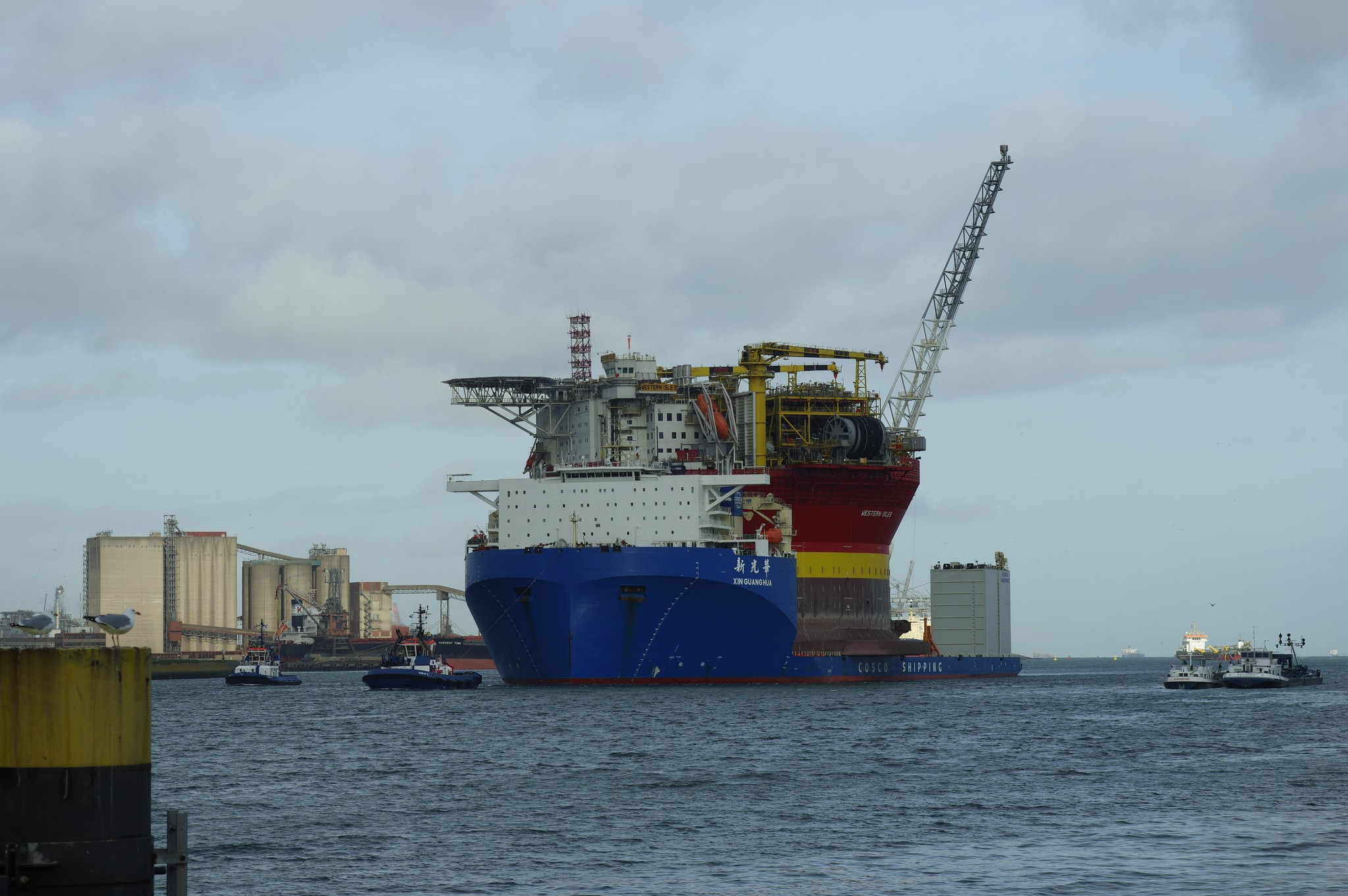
[1209,603,1216,606]
[1036,560,1041,562]
[943,543,948,544]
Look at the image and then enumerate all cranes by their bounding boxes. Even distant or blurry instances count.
[879,144,1014,470]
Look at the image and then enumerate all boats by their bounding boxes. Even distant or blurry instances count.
[1113,655,1117,660]
[1054,656,1057,661]
[1068,655,1072,659]
[225,619,303,686]
[360,604,484,692]
[1121,645,1145,658]
[442,315,1022,690]
[1164,620,1323,690]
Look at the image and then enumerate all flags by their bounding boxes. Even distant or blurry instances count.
[595,449,598,458]
[636,447,638,456]
